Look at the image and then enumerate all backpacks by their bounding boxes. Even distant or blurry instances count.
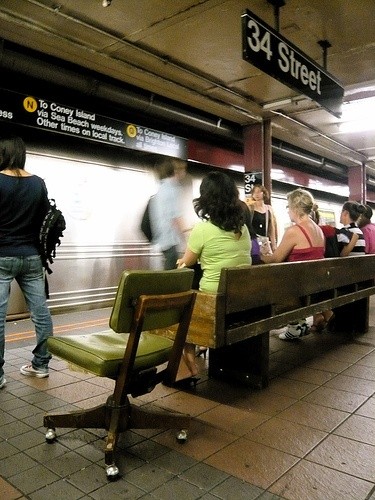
[37,178,66,274]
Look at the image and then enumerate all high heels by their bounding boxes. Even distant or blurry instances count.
[193,345,207,359]
[162,377,202,392]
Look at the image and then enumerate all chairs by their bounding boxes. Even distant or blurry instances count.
[42,263,204,479]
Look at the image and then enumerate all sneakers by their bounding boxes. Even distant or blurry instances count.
[301,320,311,335]
[0,374,6,388]
[279,325,303,340]
[20,364,49,379]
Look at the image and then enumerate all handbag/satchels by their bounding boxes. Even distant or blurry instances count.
[251,237,269,255]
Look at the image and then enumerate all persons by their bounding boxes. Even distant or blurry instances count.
[280,205,338,344]
[311,202,365,332]
[257,188,327,302]
[180,170,252,378]
[355,205,375,255]
[247,183,278,250]
[151,157,193,269]
[1,139,53,389]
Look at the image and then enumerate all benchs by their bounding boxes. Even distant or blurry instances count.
[156,251,375,391]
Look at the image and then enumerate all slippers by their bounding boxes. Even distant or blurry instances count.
[311,313,335,329]
